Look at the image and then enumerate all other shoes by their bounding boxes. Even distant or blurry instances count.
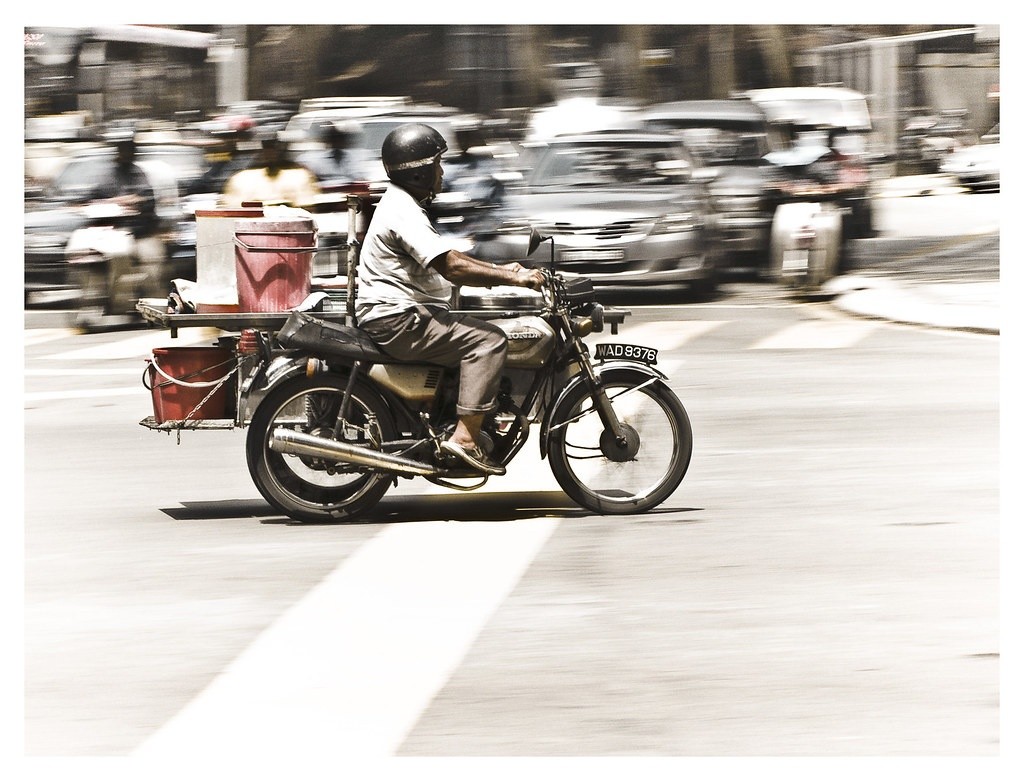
[440,441,506,477]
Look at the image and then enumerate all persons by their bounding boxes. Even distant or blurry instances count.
[357,124,548,473]
[59,116,871,319]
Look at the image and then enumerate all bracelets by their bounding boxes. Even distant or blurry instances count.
[486,262,499,289]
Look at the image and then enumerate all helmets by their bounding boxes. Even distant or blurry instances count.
[381,123,449,171]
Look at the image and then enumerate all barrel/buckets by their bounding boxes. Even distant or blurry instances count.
[142,348,227,426]
[233,218,318,313]
[194,210,265,313]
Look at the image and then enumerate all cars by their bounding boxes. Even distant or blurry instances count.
[23,82,880,303]
[903,110,1002,189]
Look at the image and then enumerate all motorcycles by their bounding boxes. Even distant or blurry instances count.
[233,231,694,517]
